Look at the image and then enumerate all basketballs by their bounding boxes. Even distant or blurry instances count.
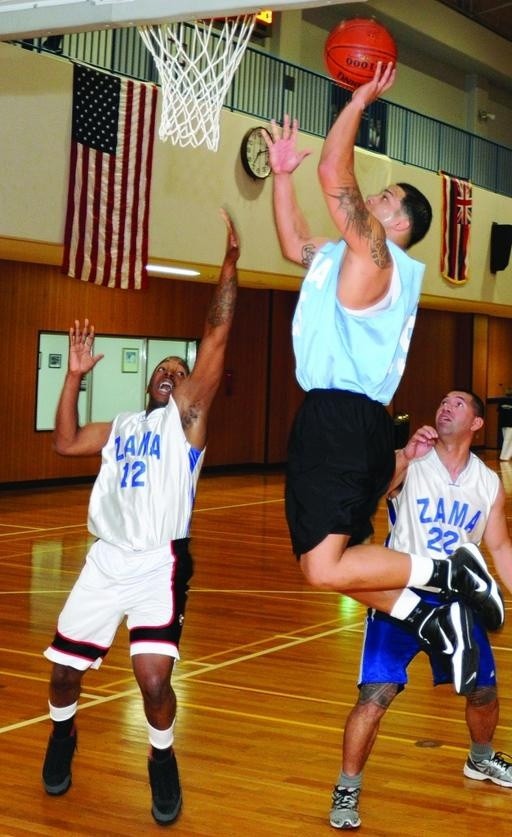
[325,19,397,91]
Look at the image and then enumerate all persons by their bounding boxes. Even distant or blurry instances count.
[38,205,243,826]
[496,388,512,461]
[328,388,512,830]
[260,56,507,697]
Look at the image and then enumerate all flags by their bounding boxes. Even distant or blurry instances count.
[59,57,158,292]
[439,167,474,284]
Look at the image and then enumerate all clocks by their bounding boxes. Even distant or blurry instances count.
[240,126,274,180]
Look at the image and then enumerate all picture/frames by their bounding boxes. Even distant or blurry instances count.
[121,348,140,373]
[49,353,62,368]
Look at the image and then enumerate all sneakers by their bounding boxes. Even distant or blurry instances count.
[41,722,80,797]
[445,542,505,634]
[462,749,512,789]
[426,600,482,697]
[146,744,184,827]
[329,777,363,831]
